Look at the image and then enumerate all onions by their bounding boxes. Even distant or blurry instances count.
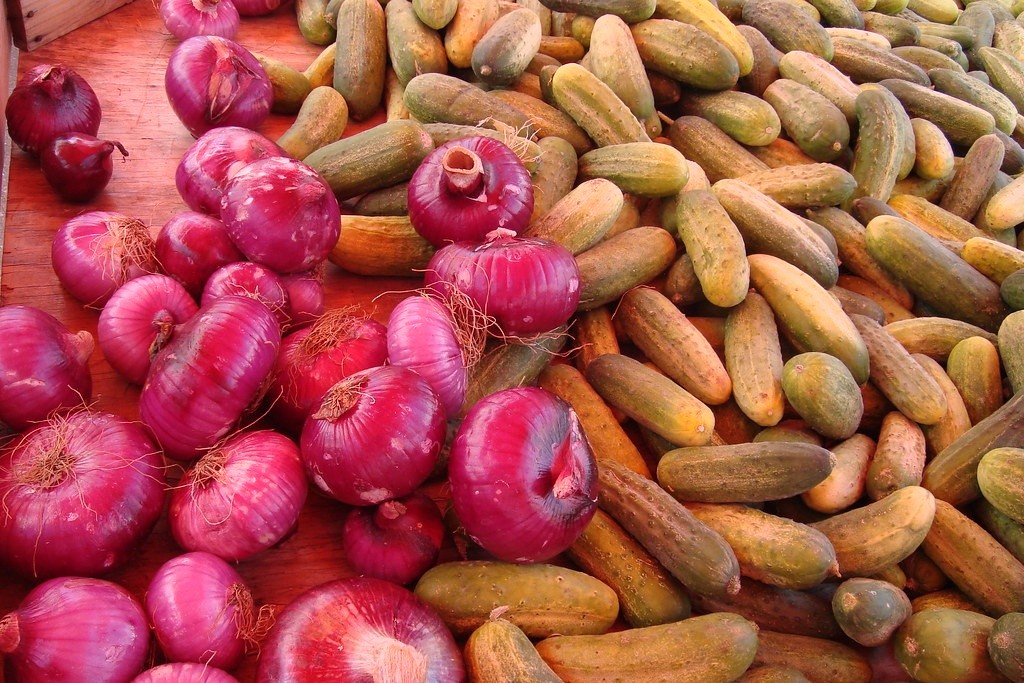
[0,0,599,683]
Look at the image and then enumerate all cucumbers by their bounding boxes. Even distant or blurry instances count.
[246,0,1024,683]
